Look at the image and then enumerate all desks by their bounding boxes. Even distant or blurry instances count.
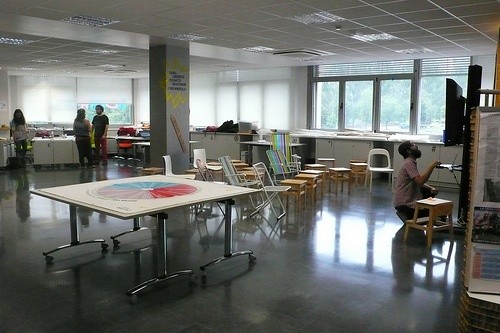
[29,174,264,305]
[31,136,89,167]
[106,135,150,169]
[238,140,306,164]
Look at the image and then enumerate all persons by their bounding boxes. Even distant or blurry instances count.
[74,110,92,168]
[79,169,93,224]
[395,225,445,296]
[96,167,107,181]
[91,106,109,165]
[16,170,29,222]
[10,108,30,170]
[395,141,442,225]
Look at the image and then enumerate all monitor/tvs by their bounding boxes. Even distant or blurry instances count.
[445,78,463,146]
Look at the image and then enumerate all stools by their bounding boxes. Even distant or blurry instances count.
[231,157,370,214]
[403,197,454,248]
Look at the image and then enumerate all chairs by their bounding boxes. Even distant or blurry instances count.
[142,167,162,176]
[266,150,296,180]
[249,161,292,221]
[269,131,302,171]
[364,148,395,194]
[162,155,196,179]
[117,127,136,158]
[217,155,265,210]
[193,148,223,181]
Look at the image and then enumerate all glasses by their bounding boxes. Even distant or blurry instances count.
[408,144,416,149]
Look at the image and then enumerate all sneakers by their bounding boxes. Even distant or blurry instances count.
[81,161,107,168]
[397,210,408,224]
